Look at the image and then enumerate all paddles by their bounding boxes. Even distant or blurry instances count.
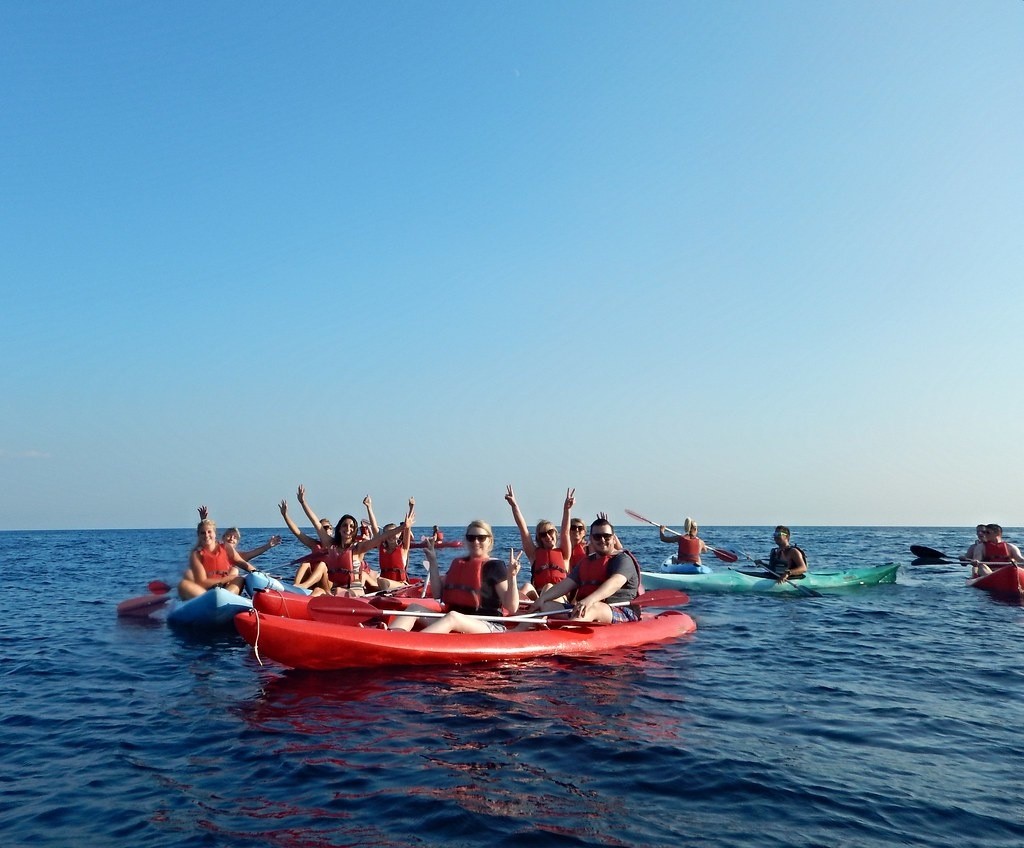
[213,569,295,581]
[420,561,430,598]
[624,508,738,562]
[510,590,689,618]
[910,545,972,561]
[148,580,178,594]
[910,556,1024,566]
[361,519,383,529]
[306,596,612,627]
[729,545,823,597]
[117,549,330,616]
[421,535,433,541]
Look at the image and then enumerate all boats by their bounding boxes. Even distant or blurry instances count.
[660,553,713,574]
[245,570,313,598]
[409,541,465,550]
[966,564,1024,602]
[640,561,899,597]
[167,586,254,635]
[258,577,534,628]
[233,611,698,667]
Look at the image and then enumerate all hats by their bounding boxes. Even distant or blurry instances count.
[229,527,240,539]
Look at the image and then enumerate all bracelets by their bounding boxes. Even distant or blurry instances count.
[785,569,792,576]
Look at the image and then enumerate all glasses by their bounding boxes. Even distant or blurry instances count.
[324,526,329,530]
[592,534,612,540]
[983,530,995,534]
[773,532,788,536]
[341,523,355,528]
[466,534,490,542]
[571,525,583,531]
[539,529,555,537]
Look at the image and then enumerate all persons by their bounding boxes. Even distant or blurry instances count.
[509,518,641,631]
[177,505,281,601]
[753,526,808,585]
[569,512,623,573]
[958,524,1024,579]
[358,520,523,633]
[660,517,707,567]
[505,484,575,603]
[430,525,443,544]
[277,484,417,594]
